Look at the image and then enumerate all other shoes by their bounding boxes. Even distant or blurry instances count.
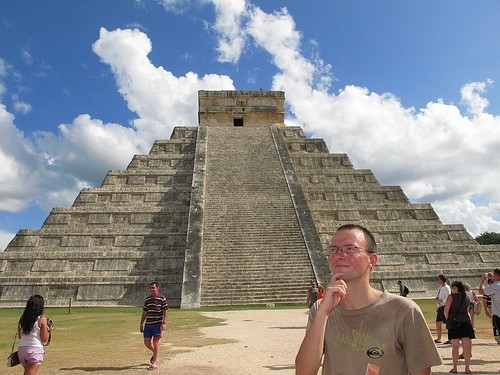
[444,341,452,344]
[434,340,441,343]
[459,354,464,359]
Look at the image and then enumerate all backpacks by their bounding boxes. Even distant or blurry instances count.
[404,286,409,294]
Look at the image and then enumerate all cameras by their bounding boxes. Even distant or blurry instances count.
[47,318,52,327]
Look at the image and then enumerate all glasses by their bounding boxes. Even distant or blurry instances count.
[329,245,373,254]
[150,287,155,289]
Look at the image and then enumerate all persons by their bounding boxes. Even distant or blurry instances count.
[443,281,476,374]
[394,269,500,375]
[295,224,442,375]
[140,282,168,371]
[307,281,325,308]
[17,295,49,375]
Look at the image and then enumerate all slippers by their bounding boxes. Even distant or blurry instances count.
[466,370,471,374]
[148,366,157,369]
[150,357,154,363]
[450,369,457,373]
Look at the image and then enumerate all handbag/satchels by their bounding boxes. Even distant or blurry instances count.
[7,333,20,367]
[446,319,455,329]
